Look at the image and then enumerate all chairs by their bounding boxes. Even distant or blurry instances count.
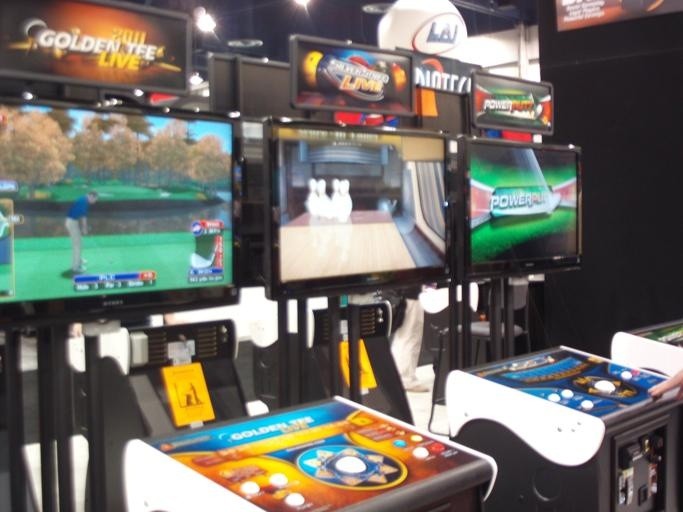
[424,270,536,440]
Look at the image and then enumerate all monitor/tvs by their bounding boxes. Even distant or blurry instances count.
[456,135,585,282]
[0,94,244,326]
[262,115,451,300]
